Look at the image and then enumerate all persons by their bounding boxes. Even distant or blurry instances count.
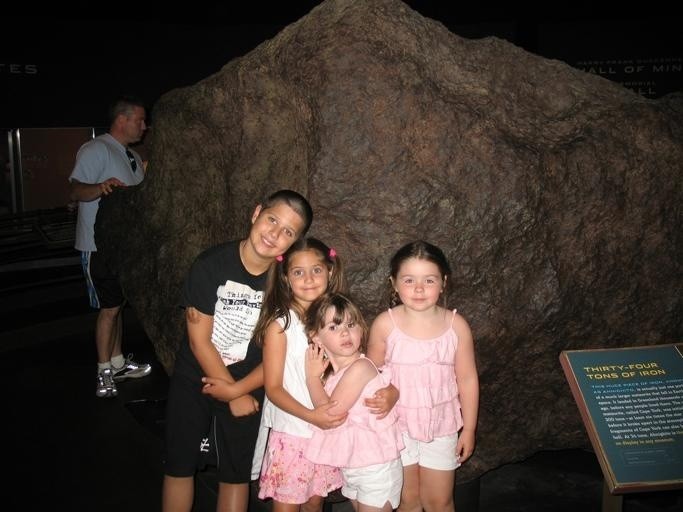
[300,290,406,511]
[154,188,314,512]
[253,238,344,512]
[366,240,480,512]
[67,98,152,399]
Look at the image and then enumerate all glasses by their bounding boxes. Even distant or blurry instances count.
[126,150,136,173]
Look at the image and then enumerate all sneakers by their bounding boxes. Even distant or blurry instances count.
[111,354,151,379]
[95,368,118,397]
